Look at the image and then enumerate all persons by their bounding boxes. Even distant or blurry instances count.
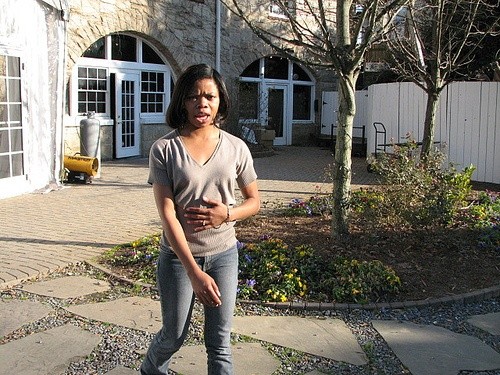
[141,65,261,375]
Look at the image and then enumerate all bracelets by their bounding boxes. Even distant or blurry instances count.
[224,204,231,222]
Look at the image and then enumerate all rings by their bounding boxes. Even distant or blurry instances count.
[202,219,205,226]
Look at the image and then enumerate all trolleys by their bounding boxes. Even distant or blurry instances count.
[367,121,388,173]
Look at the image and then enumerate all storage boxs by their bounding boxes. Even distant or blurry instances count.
[254,129,276,146]
